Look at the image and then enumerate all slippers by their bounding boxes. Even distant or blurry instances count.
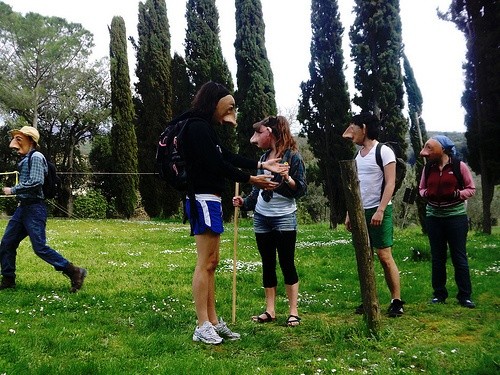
[252,312,275,323]
[286,315,301,327]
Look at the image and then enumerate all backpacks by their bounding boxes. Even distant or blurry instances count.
[155,118,205,191]
[28,149,63,202]
[352,141,407,195]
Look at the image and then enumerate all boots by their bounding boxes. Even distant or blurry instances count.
[0,274,16,290]
[62,263,87,294]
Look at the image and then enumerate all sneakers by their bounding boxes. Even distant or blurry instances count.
[193,320,225,345]
[431,295,445,303]
[460,295,476,308]
[214,317,241,341]
[388,299,405,317]
[356,300,364,314]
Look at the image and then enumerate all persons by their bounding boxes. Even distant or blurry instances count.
[418,135,477,307]
[232,115,308,326]
[0,126,87,293]
[182,81,290,344]
[342,113,403,315]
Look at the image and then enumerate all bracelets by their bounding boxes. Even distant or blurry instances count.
[260,161,262,169]
[285,175,292,184]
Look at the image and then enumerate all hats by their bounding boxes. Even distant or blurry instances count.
[7,126,40,149]
